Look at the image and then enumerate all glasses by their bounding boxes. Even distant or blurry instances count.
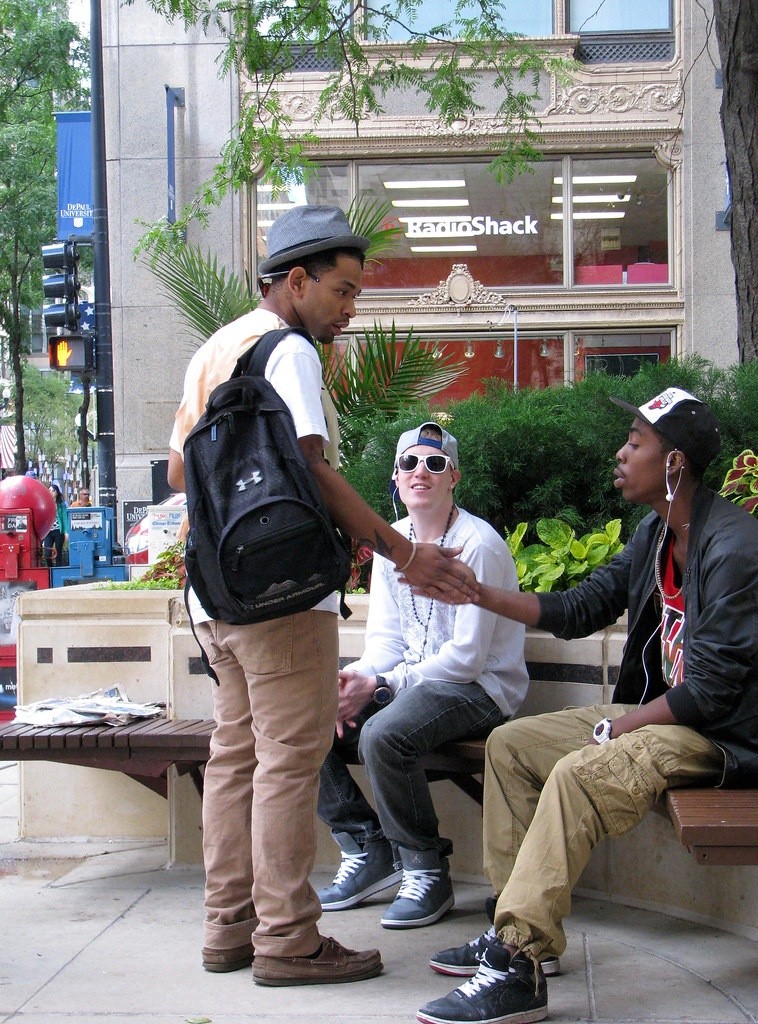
[257,268,320,298]
[397,453,455,474]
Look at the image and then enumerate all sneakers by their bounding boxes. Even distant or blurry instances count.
[316,828,401,912]
[381,846,455,930]
[415,939,548,1024]
[428,896,561,977]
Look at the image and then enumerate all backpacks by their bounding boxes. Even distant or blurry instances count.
[182,326,353,626]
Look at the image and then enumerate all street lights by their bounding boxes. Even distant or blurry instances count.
[3,386,10,416]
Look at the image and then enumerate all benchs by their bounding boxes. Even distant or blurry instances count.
[0,720,758,866]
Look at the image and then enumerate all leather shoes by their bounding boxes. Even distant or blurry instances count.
[251,937,383,987]
[202,943,255,974]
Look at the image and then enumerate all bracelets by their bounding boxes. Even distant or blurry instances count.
[394,542,417,572]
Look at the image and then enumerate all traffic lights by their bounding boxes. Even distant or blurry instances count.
[41,242,81,327]
[47,334,94,371]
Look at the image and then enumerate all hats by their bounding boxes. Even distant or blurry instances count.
[391,422,458,480]
[258,204,371,276]
[609,386,722,468]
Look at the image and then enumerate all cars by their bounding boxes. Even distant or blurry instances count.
[124,493,188,564]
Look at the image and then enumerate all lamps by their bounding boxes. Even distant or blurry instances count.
[465,341,475,358]
[574,337,580,355]
[493,340,505,358]
[432,345,442,359]
[539,339,550,357]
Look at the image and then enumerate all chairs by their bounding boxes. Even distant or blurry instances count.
[573,264,623,285]
[627,263,669,284]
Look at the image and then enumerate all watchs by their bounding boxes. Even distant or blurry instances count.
[371,675,393,711]
[593,717,612,745]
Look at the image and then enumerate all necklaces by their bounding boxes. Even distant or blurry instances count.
[656,525,683,600]
[409,504,456,658]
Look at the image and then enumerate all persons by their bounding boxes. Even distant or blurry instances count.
[318,421,528,929]
[43,484,69,567]
[71,488,93,507]
[165,203,479,988]
[390,387,757,1024]
